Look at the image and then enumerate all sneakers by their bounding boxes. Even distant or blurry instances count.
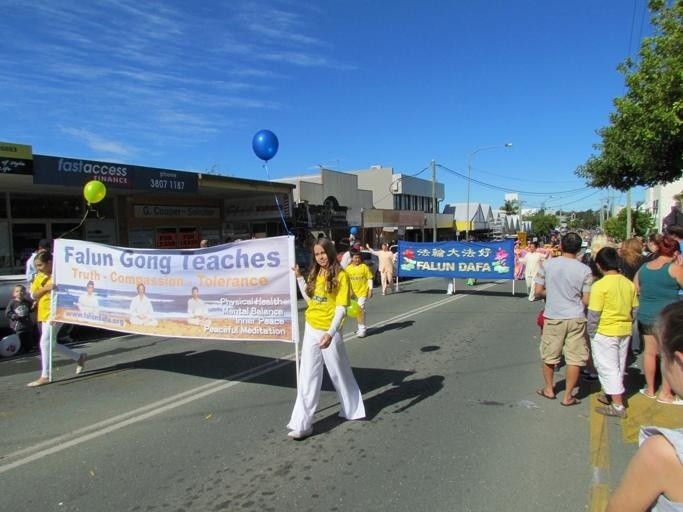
[288,426,314,439]
[356,324,367,337]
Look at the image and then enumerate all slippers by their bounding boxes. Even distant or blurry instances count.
[535,364,683,418]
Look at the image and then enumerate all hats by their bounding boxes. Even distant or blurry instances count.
[39,240,54,251]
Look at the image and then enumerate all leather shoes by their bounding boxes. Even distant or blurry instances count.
[77,353,87,374]
[27,377,51,387]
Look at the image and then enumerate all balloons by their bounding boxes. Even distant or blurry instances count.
[83,180,107,206]
[252,128,280,164]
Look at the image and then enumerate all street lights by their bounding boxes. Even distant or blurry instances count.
[542,196,555,211]
[466,143,512,241]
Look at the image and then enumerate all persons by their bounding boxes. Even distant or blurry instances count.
[514,224,682,512]
[24,239,54,355]
[128,284,158,327]
[185,287,212,329]
[4,284,38,353]
[77,280,100,322]
[26,251,87,387]
[287,233,398,439]
